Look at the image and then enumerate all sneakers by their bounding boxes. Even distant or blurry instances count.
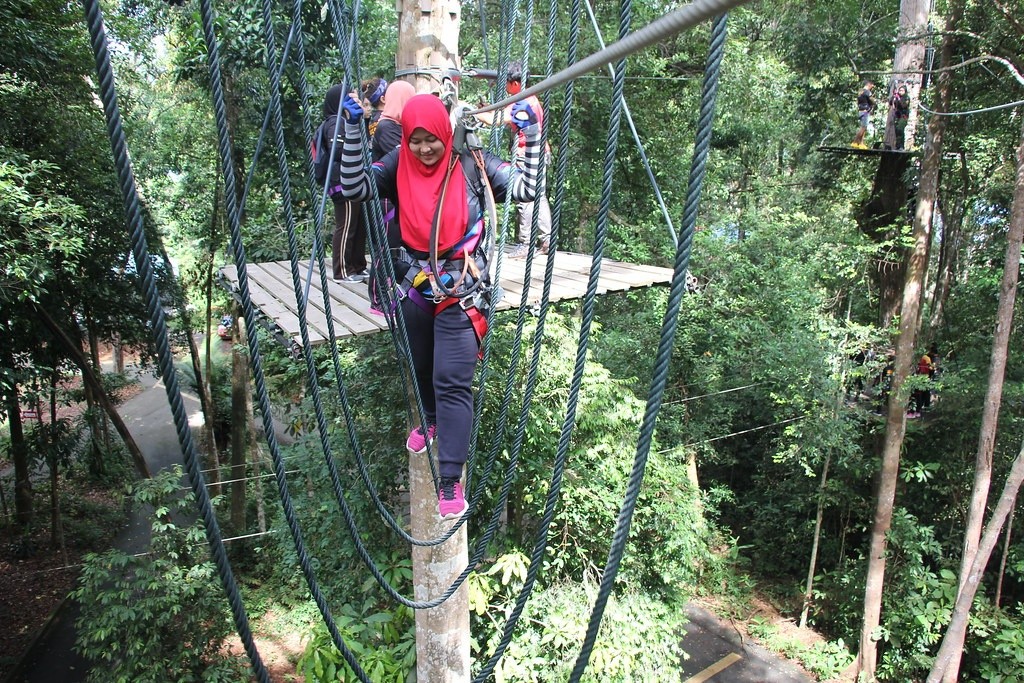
[438,483,470,520]
[333,268,370,283]
[368,304,395,317]
[508,243,537,259]
[406,419,437,454]
[536,241,550,255]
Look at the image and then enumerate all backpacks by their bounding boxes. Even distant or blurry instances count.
[312,114,345,186]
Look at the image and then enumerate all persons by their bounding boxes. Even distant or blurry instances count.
[850,80,878,149]
[846,340,939,420]
[470,64,552,258]
[315,75,416,317]
[466,67,556,243]
[889,86,910,152]
[328,81,547,521]
